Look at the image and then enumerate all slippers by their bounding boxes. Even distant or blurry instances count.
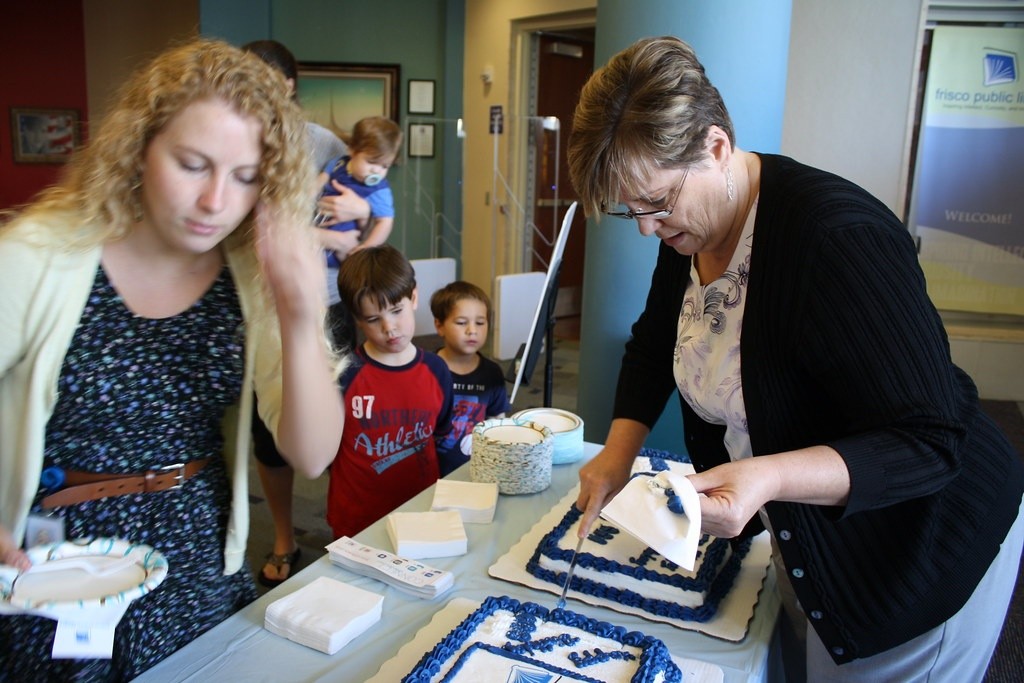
[257,546,301,589]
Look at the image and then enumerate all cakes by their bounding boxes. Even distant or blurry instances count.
[526,448,753,622]
[398,595,683,683]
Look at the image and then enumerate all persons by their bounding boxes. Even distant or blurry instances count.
[568,36,1024,683]
[0,40,510,683]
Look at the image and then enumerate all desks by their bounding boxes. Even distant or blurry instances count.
[118,443,809,683]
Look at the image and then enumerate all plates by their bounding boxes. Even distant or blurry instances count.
[510,407,584,464]
[0,537,168,614]
[472,418,551,495]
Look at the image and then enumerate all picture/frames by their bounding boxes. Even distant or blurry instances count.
[11,106,79,164]
[292,60,401,166]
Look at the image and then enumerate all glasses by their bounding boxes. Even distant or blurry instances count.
[601,162,690,219]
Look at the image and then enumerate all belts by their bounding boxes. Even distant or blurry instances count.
[40,454,215,511]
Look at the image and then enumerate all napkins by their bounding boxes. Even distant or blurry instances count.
[386,478,499,562]
[264,575,386,656]
[598,469,702,572]
